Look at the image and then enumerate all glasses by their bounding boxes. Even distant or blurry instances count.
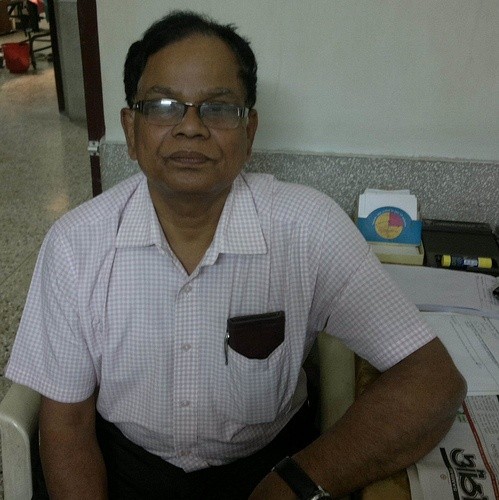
[131,97,248,130]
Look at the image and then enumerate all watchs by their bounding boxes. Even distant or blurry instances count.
[271,456,330,500]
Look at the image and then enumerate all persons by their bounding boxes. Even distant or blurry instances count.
[5,10,470,500]
